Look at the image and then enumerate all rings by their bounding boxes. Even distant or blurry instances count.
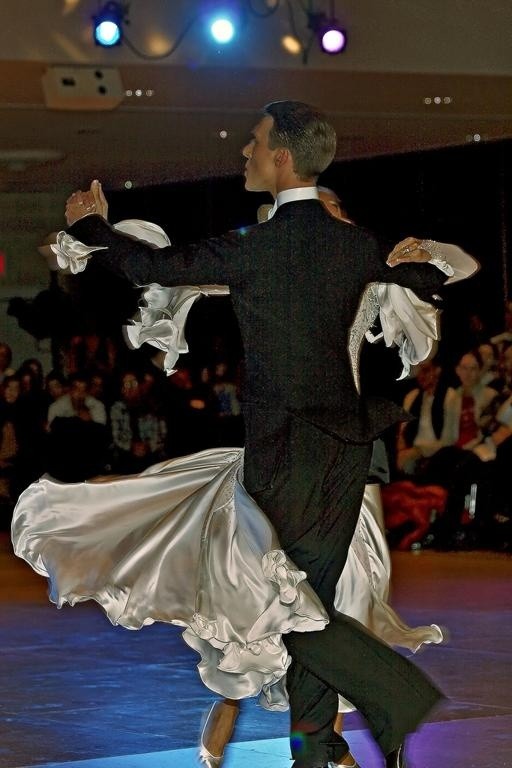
[405,246,410,255]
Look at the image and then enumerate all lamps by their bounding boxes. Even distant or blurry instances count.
[92,3,349,65]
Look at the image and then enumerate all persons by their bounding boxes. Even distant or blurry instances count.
[0,346,247,530]
[64,99,444,768]
[10,179,480,768]
[380,338,512,557]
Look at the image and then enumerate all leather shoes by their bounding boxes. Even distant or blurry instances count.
[328,738,405,768]
[197,702,223,768]
